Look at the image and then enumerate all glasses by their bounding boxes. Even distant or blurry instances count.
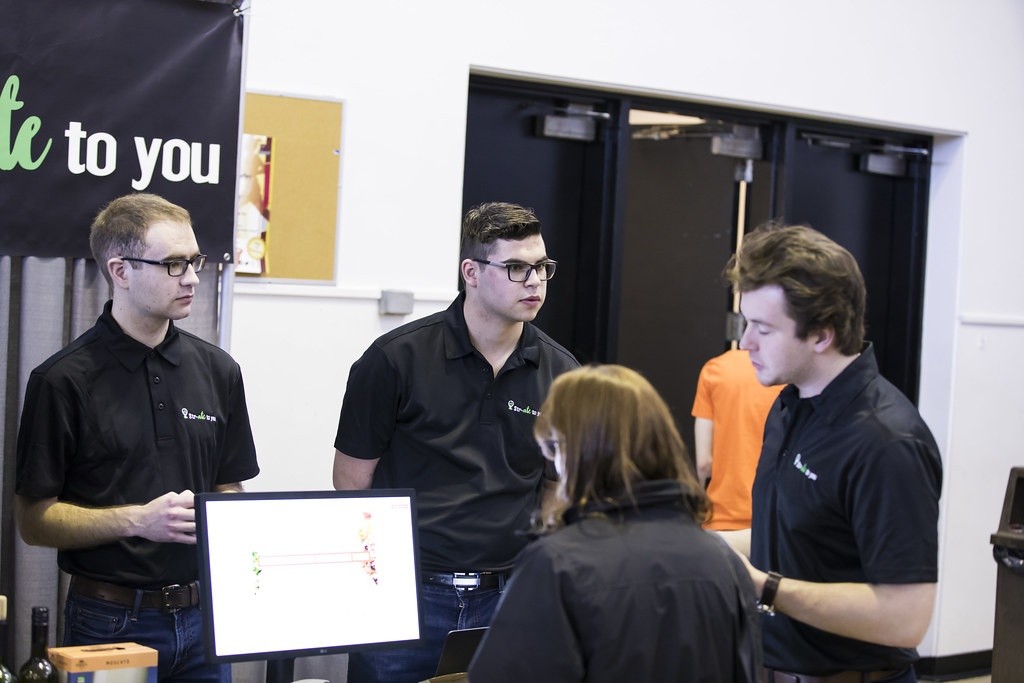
[121,254,207,277]
[472,257,558,282]
[539,435,566,458]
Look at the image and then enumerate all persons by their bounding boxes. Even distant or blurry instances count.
[1,191,261,682]
[691,220,943,683]
[331,203,589,683]
[468,363,760,682]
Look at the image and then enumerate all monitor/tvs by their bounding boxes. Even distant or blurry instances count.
[195,488,427,663]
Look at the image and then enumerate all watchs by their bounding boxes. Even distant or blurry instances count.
[755,570,785,622]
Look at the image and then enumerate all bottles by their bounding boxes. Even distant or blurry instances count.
[0,595,61,683]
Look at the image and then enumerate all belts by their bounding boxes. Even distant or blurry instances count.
[83,580,201,610]
[761,662,915,683]
[420,567,516,593]
